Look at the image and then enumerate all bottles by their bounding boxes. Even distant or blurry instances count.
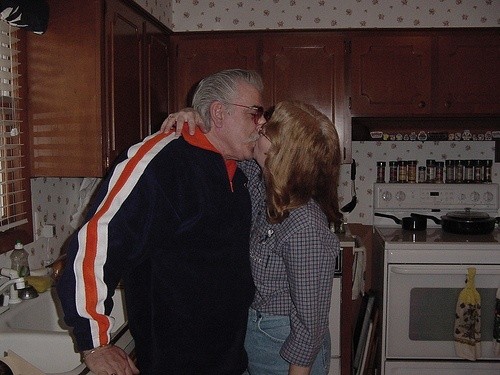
[474,160,484,184]
[408,160,417,184]
[10,240,31,277]
[445,159,455,184]
[435,162,444,183]
[398,161,407,184]
[377,162,386,183]
[465,160,474,184]
[484,160,493,183]
[455,160,464,184]
[418,167,427,183]
[389,161,398,183]
[425,160,436,184]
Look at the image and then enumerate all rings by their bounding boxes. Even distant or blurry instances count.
[169,116,176,122]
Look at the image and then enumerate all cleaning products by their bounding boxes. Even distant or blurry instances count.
[10,239,30,290]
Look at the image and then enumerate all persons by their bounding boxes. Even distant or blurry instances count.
[57,69,267,375]
[160,100,341,375]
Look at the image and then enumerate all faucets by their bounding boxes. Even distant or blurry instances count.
[0,268,23,305]
[0,277,26,296]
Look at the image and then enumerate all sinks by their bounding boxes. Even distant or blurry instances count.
[0,333,87,375]
[4,288,125,333]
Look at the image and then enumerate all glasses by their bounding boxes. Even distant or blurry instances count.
[258,126,272,145]
[228,103,264,125]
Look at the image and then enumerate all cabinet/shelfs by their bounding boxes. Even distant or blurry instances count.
[29,0,500,179]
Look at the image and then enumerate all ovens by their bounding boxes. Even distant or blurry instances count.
[384,263,500,375]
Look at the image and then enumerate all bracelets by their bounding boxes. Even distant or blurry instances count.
[82,344,105,359]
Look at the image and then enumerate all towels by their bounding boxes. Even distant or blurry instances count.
[450,268,482,363]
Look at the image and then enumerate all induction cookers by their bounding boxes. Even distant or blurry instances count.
[374,182,500,264]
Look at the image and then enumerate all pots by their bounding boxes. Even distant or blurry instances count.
[374,212,427,230]
[410,207,500,236]
